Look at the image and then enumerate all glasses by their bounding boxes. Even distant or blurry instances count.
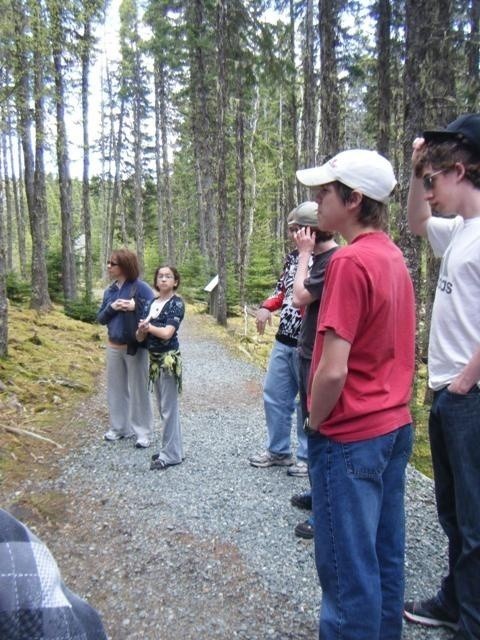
[107,260,119,267]
[423,165,453,189]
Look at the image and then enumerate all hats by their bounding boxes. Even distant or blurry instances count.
[295,147,398,205]
[421,112,480,155]
[286,200,320,227]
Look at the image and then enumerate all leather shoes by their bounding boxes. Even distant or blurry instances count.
[151,453,160,460]
[151,459,175,470]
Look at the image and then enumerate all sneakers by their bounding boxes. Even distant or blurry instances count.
[403,595,462,631]
[288,461,309,477]
[291,489,312,509]
[248,451,295,468]
[135,436,153,448]
[103,429,134,440]
[296,520,314,538]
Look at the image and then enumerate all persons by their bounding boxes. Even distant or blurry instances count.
[97,249,155,447]
[0,507,109,640]
[136,264,185,471]
